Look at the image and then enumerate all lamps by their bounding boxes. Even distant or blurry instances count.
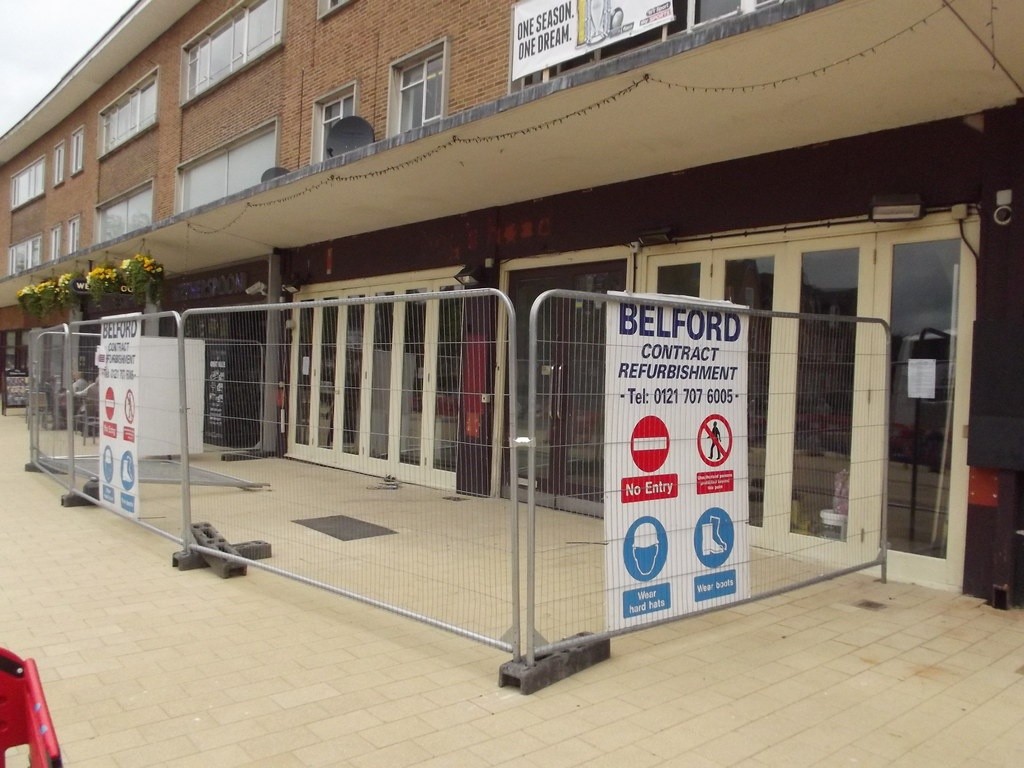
[453,262,486,289]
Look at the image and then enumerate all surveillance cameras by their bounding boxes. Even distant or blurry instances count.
[994,206,1012,225]
[246,282,267,296]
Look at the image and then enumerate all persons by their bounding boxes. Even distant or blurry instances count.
[43,370,98,437]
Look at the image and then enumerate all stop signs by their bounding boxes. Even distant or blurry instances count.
[104,387,117,420]
[629,415,670,474]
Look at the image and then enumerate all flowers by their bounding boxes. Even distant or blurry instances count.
[54,273,84,305]
[86,264,122,308]
[120,255,166,306]
[16,283,39,315]
[34,277,57,312]
